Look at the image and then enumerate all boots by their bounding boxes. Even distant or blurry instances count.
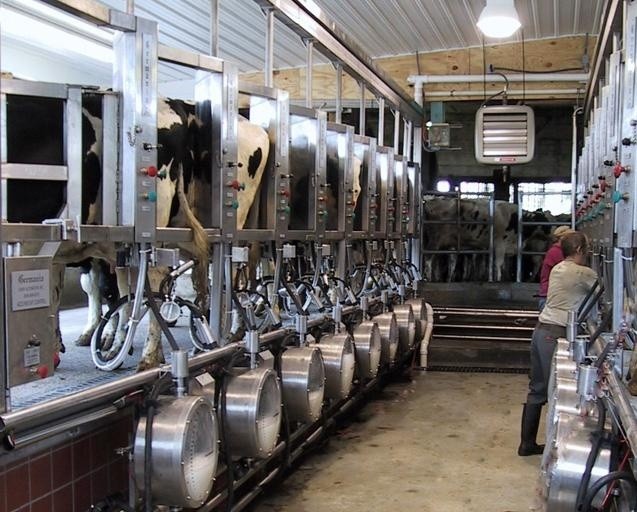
[517,399,550,458]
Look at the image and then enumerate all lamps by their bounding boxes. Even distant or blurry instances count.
[476,0,521,38]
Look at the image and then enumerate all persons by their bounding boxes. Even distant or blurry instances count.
[537,225,575,314]
[518,231,602,456]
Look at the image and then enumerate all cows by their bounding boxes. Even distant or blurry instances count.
[422,194,572,283]
[4,93,270,374]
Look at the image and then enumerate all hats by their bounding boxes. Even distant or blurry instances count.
[553,225,573,237]
